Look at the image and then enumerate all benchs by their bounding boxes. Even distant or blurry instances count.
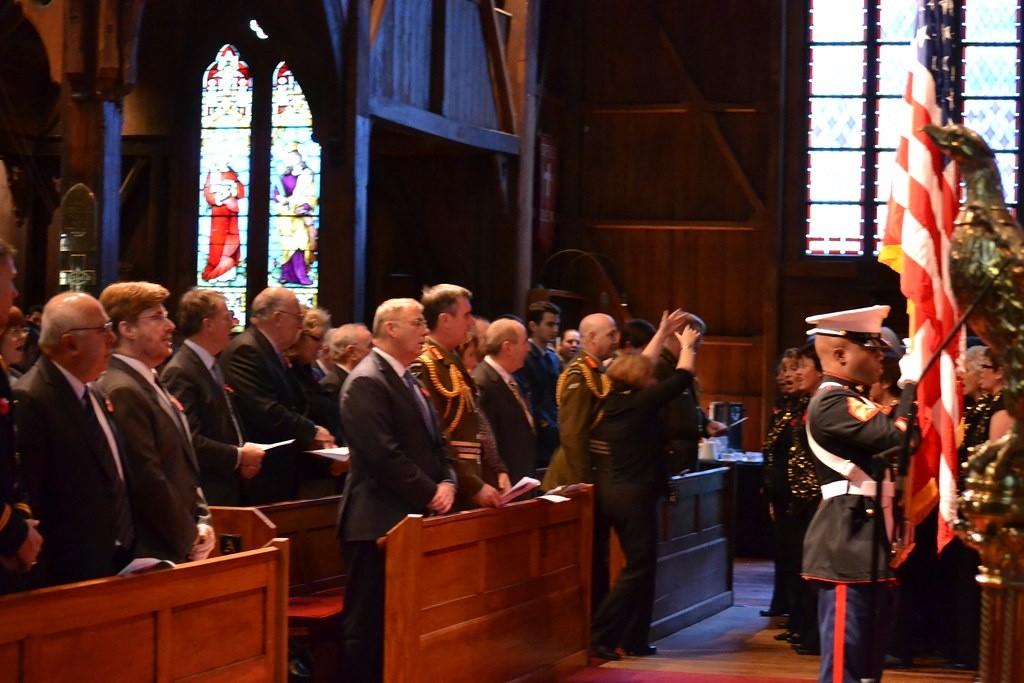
[289,584,346,683]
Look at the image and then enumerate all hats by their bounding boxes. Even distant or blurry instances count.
[804,301,896,349]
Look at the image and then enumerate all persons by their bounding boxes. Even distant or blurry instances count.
[591,307,702,662]
[0,243,42,590]
[219,287,337,679]
[406,282,543,508]
[279,301,372,477]
[337,297,458,683]
[759,304,1015,683]
[10,292,140,586]
[160,289,265,479]
[96,282,215,561]
[511,301,728,494]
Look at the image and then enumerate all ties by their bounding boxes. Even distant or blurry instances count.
[509,379,534,429]
[404,370,433,442]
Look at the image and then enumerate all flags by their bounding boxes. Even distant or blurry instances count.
[878,0,966,571]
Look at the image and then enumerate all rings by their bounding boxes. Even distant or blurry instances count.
[31,561,37,565]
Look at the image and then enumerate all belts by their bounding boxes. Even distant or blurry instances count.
[820,480,896,501]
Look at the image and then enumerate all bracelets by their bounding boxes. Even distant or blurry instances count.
[680,343,694,350]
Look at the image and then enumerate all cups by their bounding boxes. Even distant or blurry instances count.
[702,441,721,459]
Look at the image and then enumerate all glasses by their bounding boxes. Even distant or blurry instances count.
[5,325,32,339]
[63,321,119,335]
[274,306,304,324]
[391,315,432,328]
[978,361,994,372]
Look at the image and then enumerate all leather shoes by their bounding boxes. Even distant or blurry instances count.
[627,641,659,658]
[592,635,619,662]
[774,627,819,656]
[752,604,790,616]
